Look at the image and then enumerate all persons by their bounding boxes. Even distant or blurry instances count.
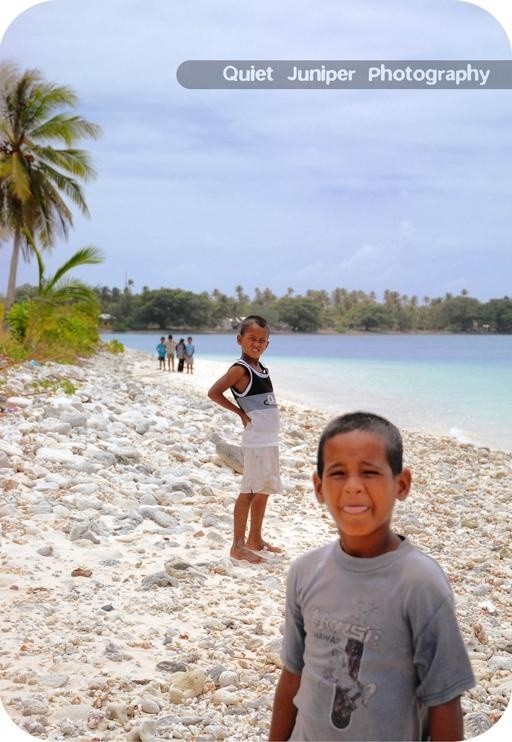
[164,334,179,373]
[206,314,285,565]
[268,410,478,742]
[155,336,167,370]
[184,336,195,374]
[174,338,187,372]
